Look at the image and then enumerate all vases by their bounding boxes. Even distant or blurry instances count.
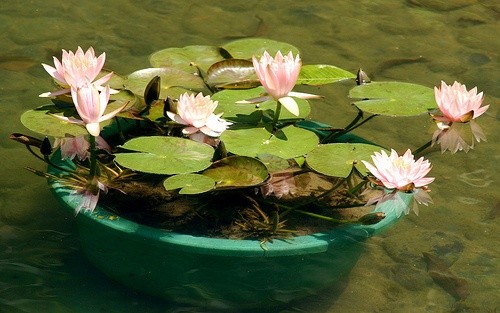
[41,118,420,312]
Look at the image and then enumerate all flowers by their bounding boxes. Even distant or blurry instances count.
[6,35,492,241]
